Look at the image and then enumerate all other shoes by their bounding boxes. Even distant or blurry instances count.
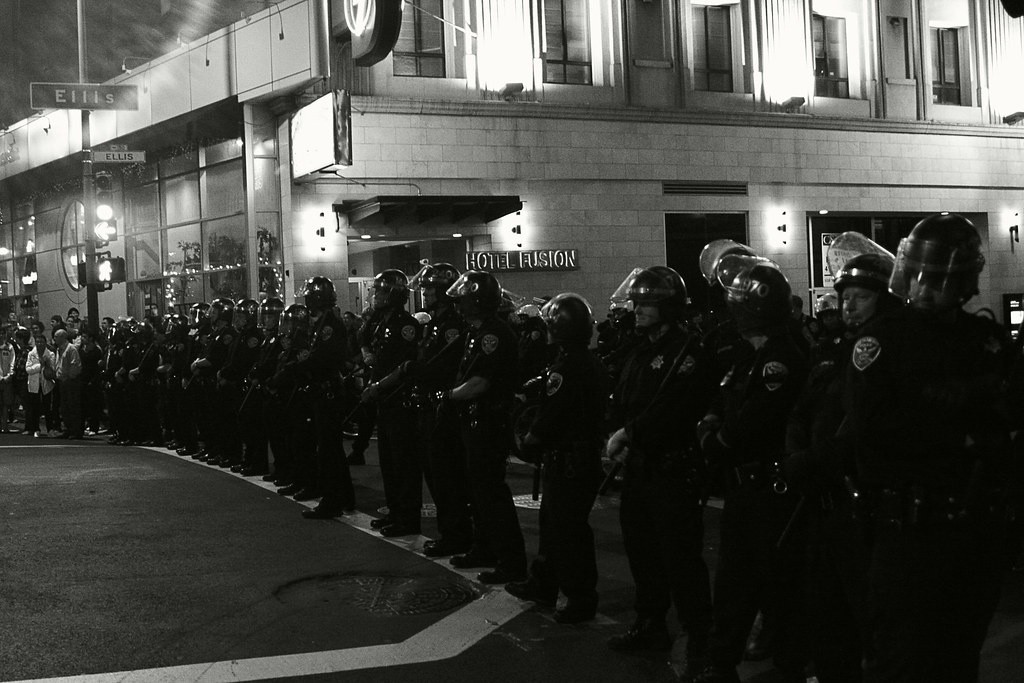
[0,414,772,683]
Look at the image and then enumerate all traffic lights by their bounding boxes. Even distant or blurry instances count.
[97,256,128,283]
[94,172,119,243]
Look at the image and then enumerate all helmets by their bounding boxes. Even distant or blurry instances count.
[167,313,191,336]
[903,214,985,302]
[372,269,410,310]
[210,298,235,321]
[305,276,337,317]
[708,247,755,296]
[517,303,540,319]
[417,262,459,303]
[833,253,904,318]
[190,302,211,329]
[257,298,285,328]
[233,298,259,331]
[814,293,838,313]
[450,269,502,321]
[412,311,432,324]
[544,292,592,347]
[284,304,311,334]
[726,266,791,338]
[630,265,687,320]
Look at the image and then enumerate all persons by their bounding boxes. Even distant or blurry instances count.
[832,213,1024,683]
[340,265,607,623]
[0,275,349,520]
[597,233,898,683]
